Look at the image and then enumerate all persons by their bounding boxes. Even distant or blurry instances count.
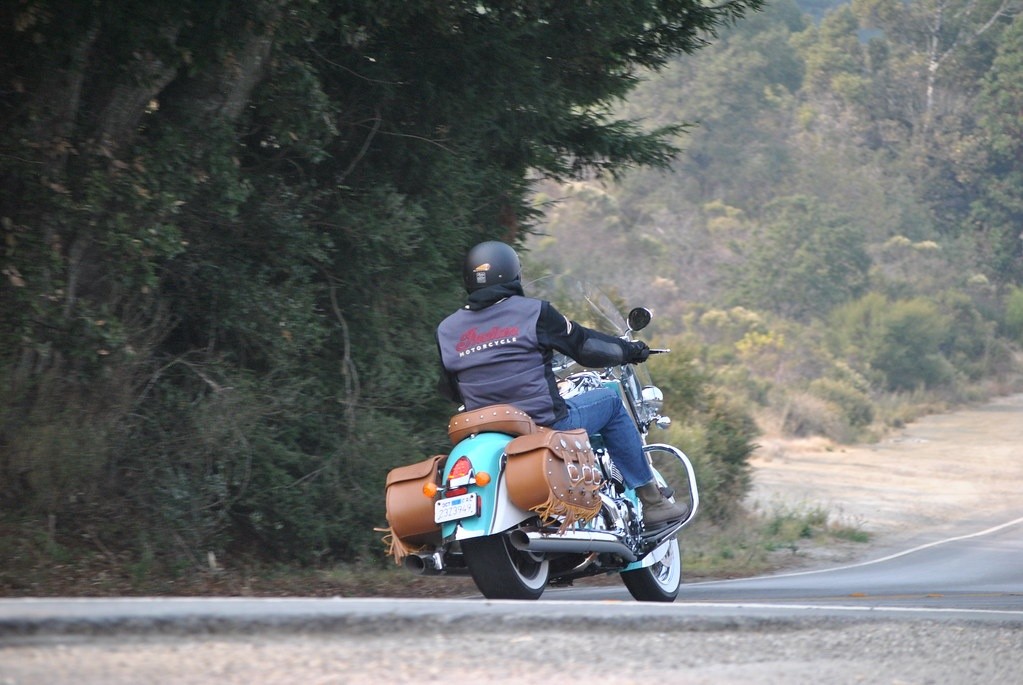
[432,239,689,531]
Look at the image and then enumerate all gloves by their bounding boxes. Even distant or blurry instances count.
[630,340,649,365]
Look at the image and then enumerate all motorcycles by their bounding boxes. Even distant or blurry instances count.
[380,272,701,603]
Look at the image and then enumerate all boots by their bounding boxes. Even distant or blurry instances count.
[635,481,690,532]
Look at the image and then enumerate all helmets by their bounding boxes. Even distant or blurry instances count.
[462,241,521,295]
